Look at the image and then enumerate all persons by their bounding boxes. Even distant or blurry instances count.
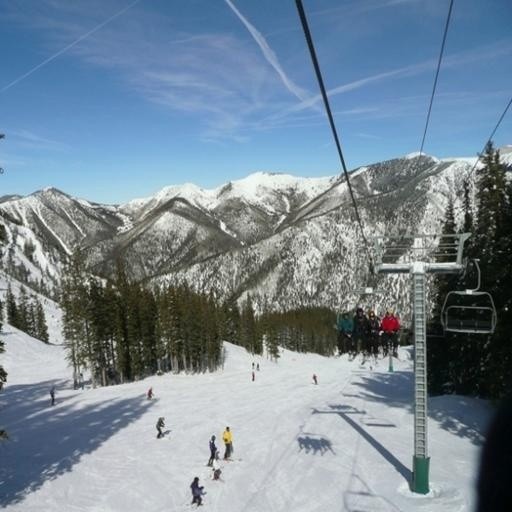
[337,311,356,355]
[147,385,156,400]
[190,476,207,505]
[354,308,367,354]
[381,306,401,358]
[208,435,216,466]
[312,372,318,386]
[156,416,166,437]
[367,309,379,358]
[223,426,232,459]
[51,387,55,405]
